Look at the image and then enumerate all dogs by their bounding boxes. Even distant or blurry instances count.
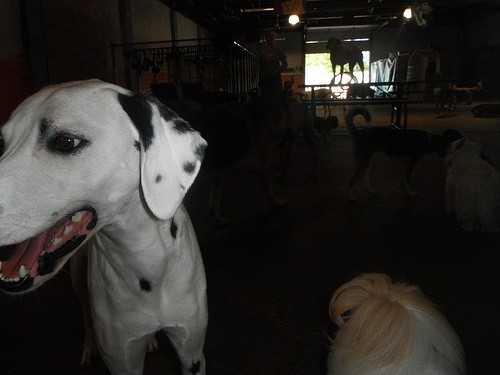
[209,83,500,234]
[0,78,208,370]
[325,37,364,86]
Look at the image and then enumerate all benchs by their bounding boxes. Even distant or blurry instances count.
[300,78,419,133]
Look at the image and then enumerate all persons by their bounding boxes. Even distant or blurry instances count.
[250,30,289,99]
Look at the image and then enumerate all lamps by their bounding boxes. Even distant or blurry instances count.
[288,14,300,26]
[404,8,413,19]
